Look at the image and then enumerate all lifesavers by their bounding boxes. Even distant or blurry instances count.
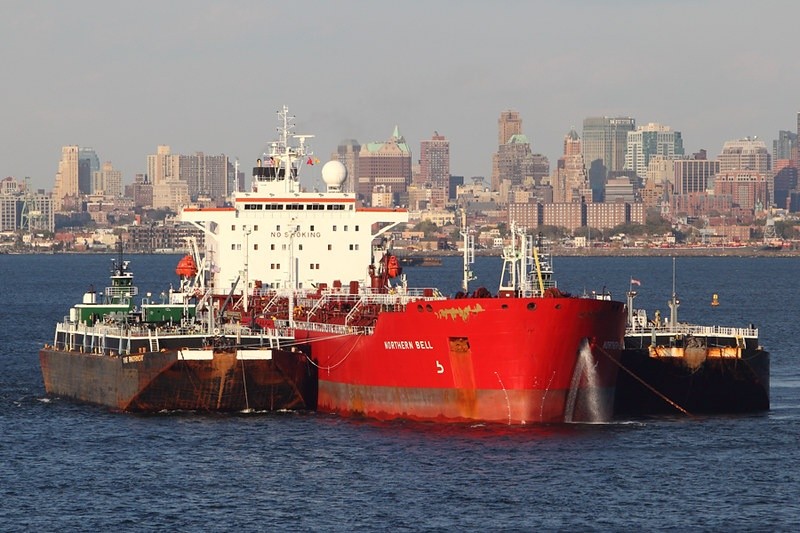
[213,328,220,335]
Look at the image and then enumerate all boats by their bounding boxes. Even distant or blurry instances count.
[39,104,771,424]
[400,255,442,267]
